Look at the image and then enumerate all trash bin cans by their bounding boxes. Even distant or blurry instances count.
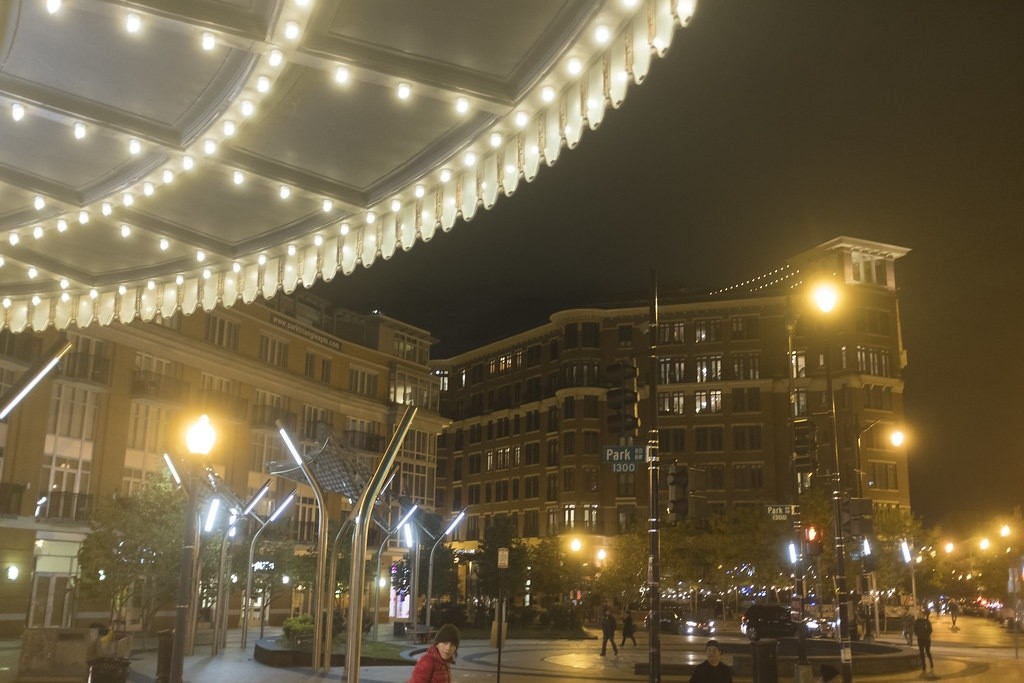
[87,657,130,683]
[751,638,780,683]
[154,630,173,679]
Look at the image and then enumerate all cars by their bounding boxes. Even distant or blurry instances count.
[644,608,715,636]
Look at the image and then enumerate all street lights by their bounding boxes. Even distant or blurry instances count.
[786,268,844,683]
[569,533,608,636]
[854,412,910,640]
[275,402,470,683]
[164,404,299,683]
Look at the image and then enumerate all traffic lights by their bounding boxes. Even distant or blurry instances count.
[794,419,819,474]
[606,363,642,434]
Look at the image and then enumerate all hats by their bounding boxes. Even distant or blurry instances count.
[821,664,838,681]
[706,640,723,656]
[434,624,459,648]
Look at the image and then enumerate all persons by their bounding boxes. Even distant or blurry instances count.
[914,611,934,671]
[820,664,841,683]
[600,609,618,656]
[406,624,460,683]
[902,607,916,646]
[950,602,958,626]
[620,611,637,648]
[687,640,734,683]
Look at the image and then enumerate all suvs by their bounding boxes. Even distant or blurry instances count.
[741,606,837,640]
